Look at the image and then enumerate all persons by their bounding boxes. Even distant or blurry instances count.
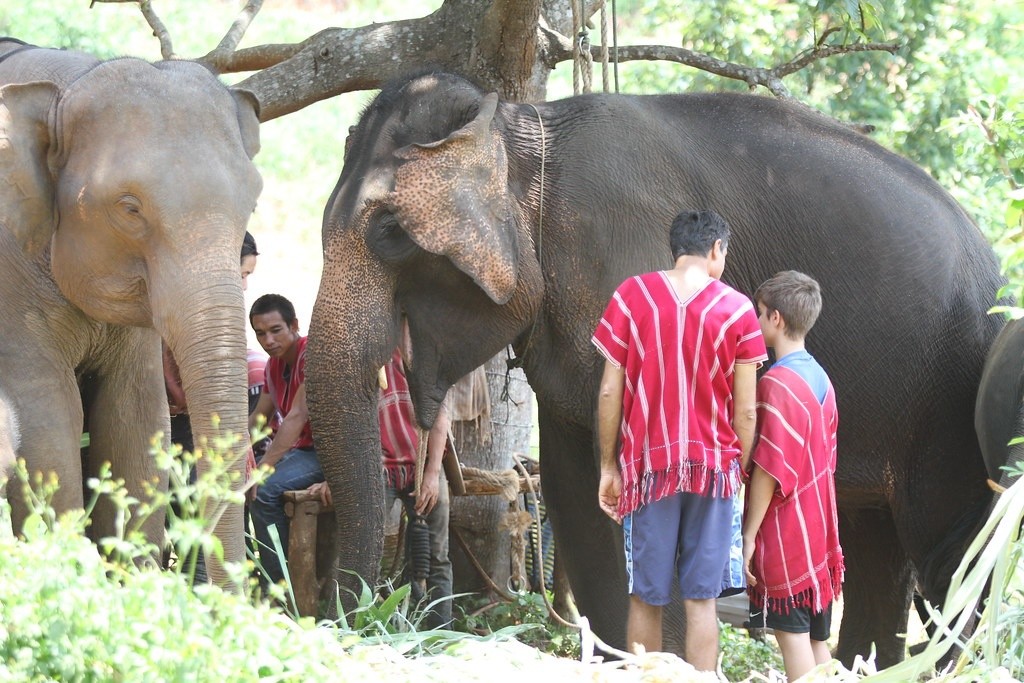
[162,232,259,414]
[742,270,845,683]
[248,294,326,598]
[309,345,452,631]
[247,348,269,415]
[590,209,769,672]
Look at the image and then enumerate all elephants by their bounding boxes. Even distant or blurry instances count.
[0,37,263,597]
[306,63,1023,679]
